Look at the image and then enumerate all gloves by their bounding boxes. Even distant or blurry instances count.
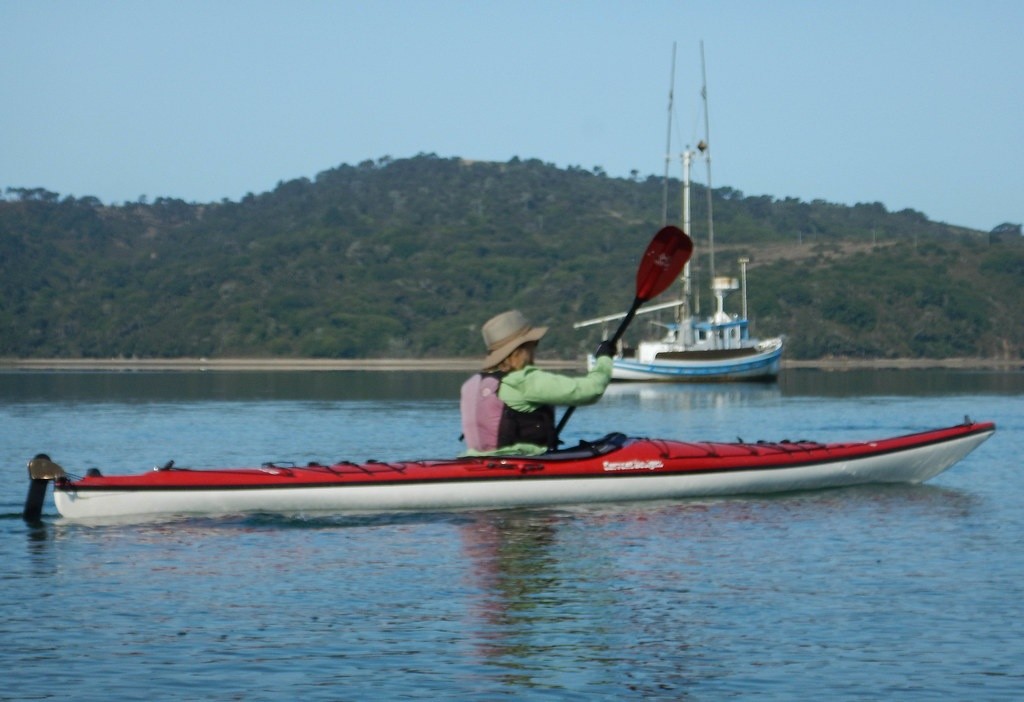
[594,340,617,359]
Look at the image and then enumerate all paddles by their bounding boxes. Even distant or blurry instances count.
[555,225,696,433]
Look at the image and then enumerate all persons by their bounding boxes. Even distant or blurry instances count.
[480,309,617,458]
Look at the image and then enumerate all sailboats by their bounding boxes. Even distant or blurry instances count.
[568,38,786,385]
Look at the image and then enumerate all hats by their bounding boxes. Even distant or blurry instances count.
[478,309,549,371]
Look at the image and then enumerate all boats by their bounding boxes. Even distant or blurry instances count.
[23,420,997,528]
